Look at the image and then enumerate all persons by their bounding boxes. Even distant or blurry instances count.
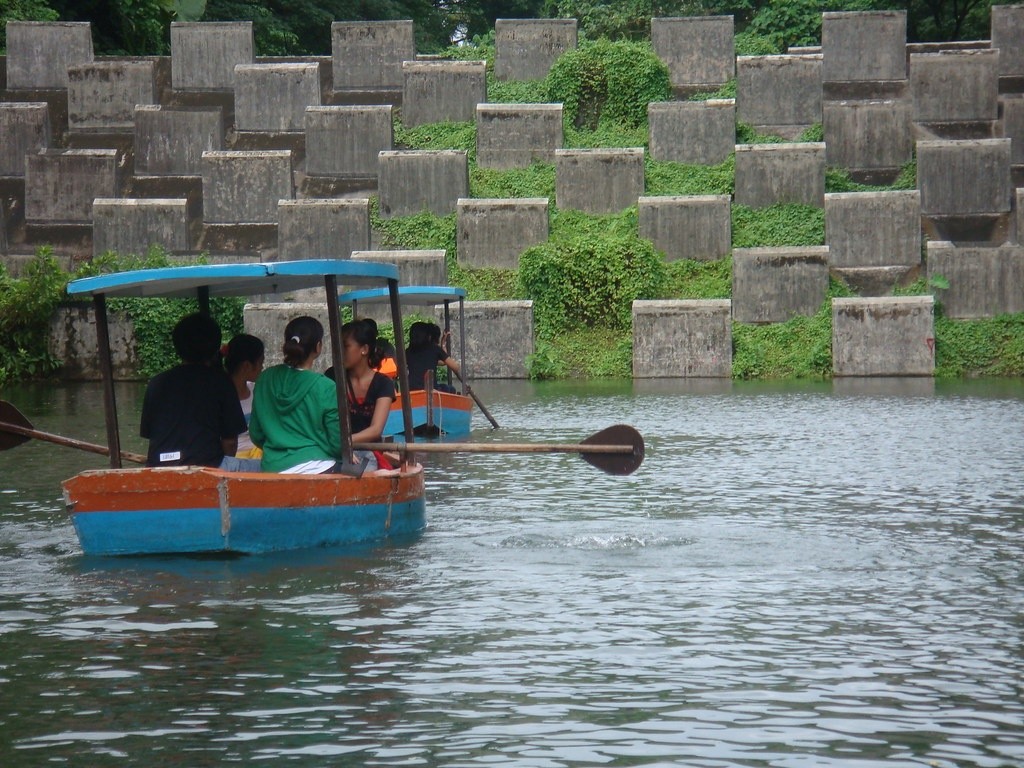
[405,322,459,394]
[248,316,377,474]
[140,313,261,472]
[220,333,265,459]
[363,318,399,394]
[324,321,397,456]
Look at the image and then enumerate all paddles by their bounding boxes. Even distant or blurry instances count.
[0,400,147,464]
[353,424,646,475]
[455,371,500,430]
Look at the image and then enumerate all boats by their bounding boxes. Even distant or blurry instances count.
[57,258,426,562]
[340,286,475,446]
